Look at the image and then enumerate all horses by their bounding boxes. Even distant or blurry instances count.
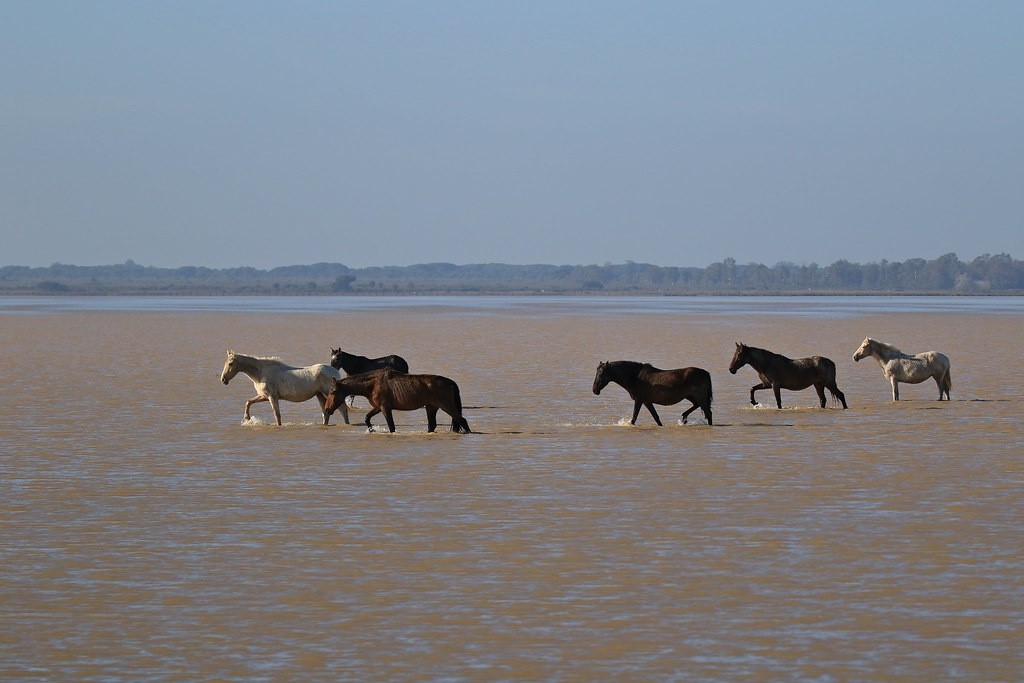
[729,341,850,410]
[216,344,475,434]
[591,358,716,427]
[852,334,955,404]
